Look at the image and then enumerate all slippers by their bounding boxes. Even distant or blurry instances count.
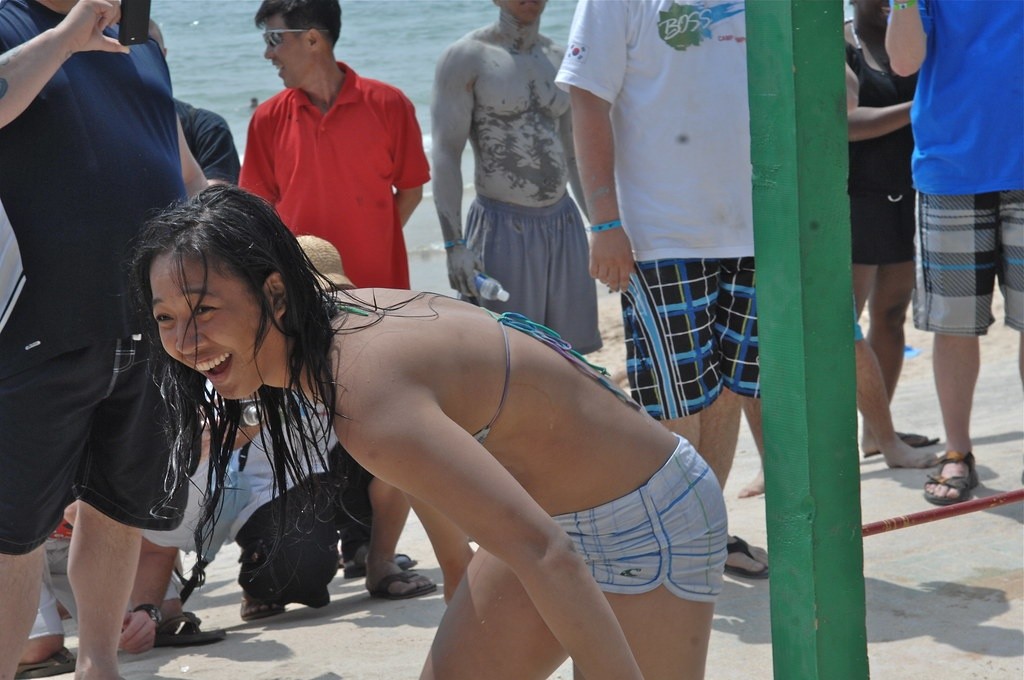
[150,610,227,646]
[722,535,771,579]
[862,431,941,459]
[343,553,417,579]
[239,592,286,621]
[371,569,437,600]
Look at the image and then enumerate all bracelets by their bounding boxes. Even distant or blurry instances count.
[589,219,622,231]
[444,239,465,249]
[893,0,917,10]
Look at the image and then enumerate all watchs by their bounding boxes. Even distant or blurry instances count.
[133,603,162,622]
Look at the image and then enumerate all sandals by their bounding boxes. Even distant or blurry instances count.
[923,449,979,505]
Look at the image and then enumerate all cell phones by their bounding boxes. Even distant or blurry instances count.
[118,0,151,46]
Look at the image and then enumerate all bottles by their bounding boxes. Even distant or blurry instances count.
[470,269,511,304]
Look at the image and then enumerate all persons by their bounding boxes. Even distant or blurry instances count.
[552,0,769,577]
[842,0,941,458]
[738,292,941,501]
[430,0,602,355]
[884,0,1024,505]
[0,0,432,680]
[120,186,728,680]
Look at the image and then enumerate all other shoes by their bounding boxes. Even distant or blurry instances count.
[15,647,76,679]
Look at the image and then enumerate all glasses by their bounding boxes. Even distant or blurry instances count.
[263,29,327,47]
